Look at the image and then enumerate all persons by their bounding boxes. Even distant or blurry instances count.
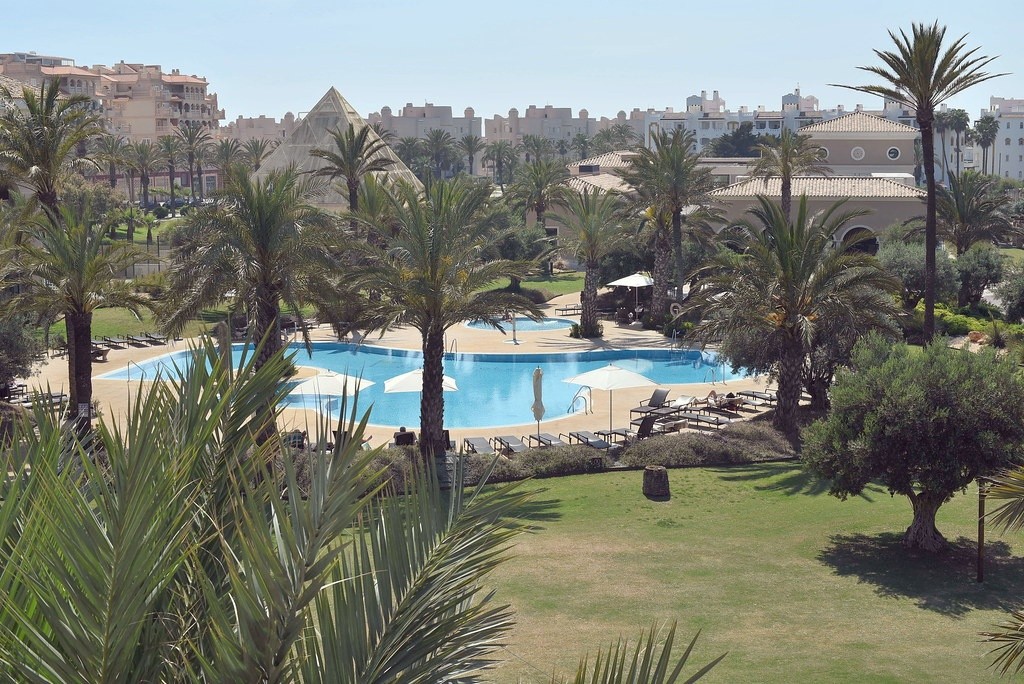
[399,427,406,432]
[362,435,372,443]
[694,390,735,408]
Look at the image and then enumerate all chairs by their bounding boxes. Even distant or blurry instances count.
[555,304,633,325]
[443,388,778,458]
[332,431,371,450]
[389,432,417,448]
[53,330,168,361]
[281,432,308,451]
[0,382,68,408]
[310,442,334,455]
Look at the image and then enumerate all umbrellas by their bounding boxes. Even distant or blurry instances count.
[606,273,654,321]
